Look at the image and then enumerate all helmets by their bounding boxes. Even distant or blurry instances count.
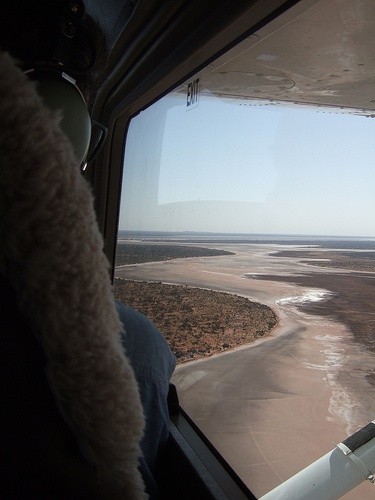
[16,63,90,169]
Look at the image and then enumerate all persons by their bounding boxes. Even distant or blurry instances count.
[1,63,173,499]
